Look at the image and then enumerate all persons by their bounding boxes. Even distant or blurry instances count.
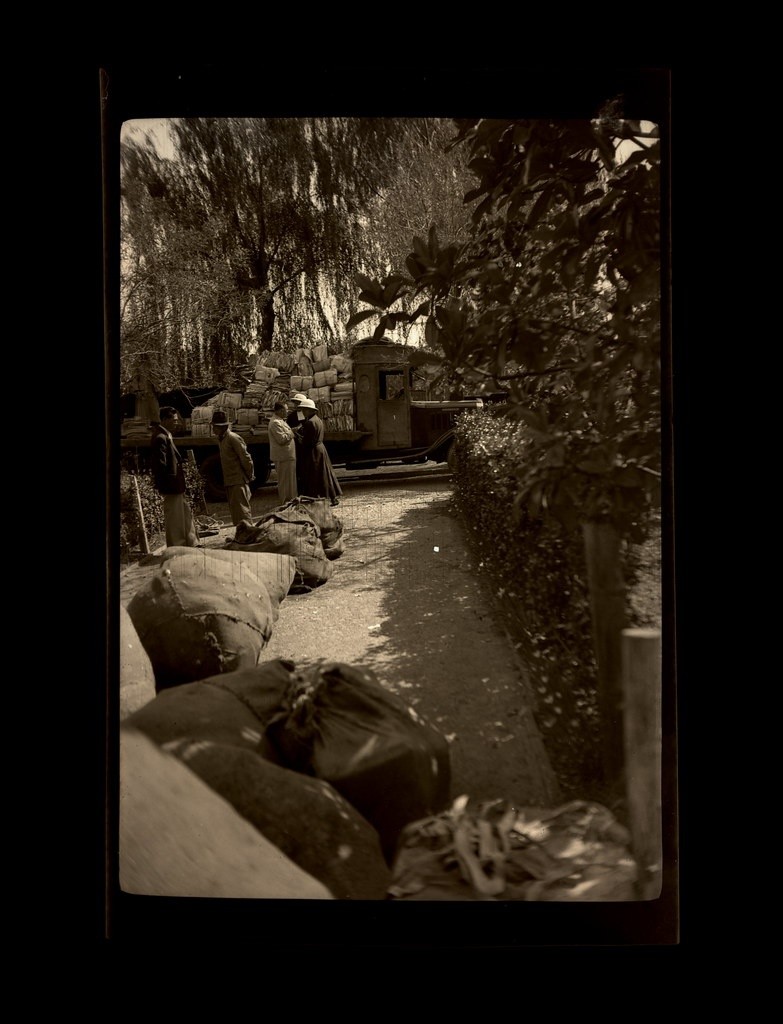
[297,398,344,506]
[207,411,256,529]
[149,406,202,551]
[286,393,307,440]
[268,402,299,505]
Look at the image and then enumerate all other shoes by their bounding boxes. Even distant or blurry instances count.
[331,498,339,505]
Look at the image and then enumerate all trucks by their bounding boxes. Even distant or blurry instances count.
[115,343,487,503]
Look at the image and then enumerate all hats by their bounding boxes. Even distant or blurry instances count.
[207,411,232,425]
[296,399,318,410]
[290,393,306,403]
